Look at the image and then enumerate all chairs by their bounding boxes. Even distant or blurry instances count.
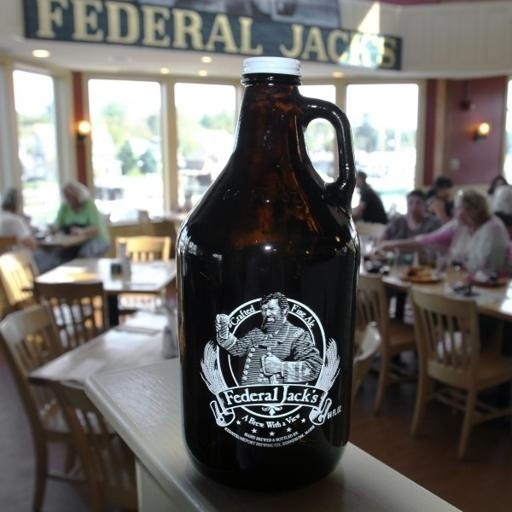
[29,277,112,339]
[0,302,139,505]
[0,247,44,307]
[117,235,171,261]
[409,285,510,453]
[349,271,419,412]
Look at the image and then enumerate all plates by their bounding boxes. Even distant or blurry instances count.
[475,278,506,288]
[399,272,441,284]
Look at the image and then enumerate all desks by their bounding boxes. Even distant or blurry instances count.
[37,255,191,324]
[32,309,188,408]
[354,253,512,391]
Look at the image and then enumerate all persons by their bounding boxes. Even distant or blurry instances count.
[486,175,508,195]
[216,292,322,384]
[349,171,389,223]
[2,187,36,254]
[491,185,512,239]
[38,183,109,256]
[365,190,445,314]
[423,176,452,218]
[373,188,512,348]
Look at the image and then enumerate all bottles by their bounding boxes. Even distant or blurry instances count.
[172,54,360,491]
[116,240,131,275]
